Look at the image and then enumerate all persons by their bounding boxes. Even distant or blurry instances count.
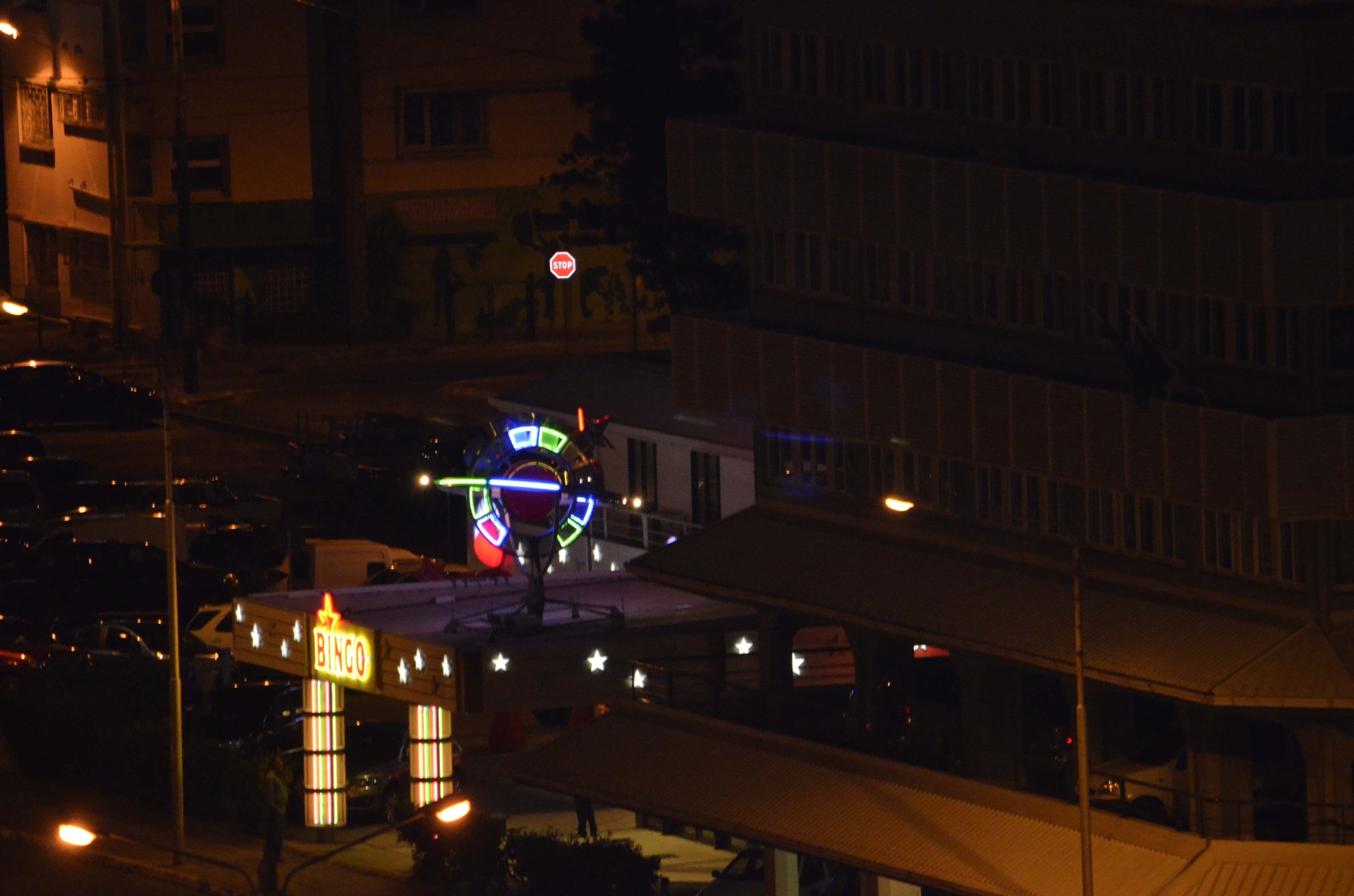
[571,793,599,837]
[265,757,289,864]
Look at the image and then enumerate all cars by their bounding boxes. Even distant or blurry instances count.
[1,613,98,669]
[48,620,220,661]
[1,355,160,432]
[1,413,478,614]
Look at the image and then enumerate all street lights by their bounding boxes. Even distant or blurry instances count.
[1,297,186,868]
[53,793,474,896]
[881,492,1091,895]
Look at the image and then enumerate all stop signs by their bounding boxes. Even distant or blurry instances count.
[549,251,576,279]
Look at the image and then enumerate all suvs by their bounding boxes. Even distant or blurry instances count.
[187,603,234,651]
